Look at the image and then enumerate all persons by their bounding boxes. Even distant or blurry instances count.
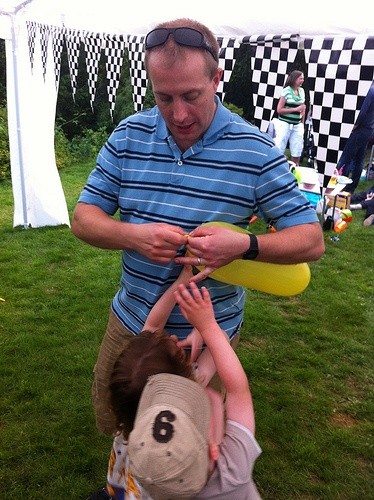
[336,81,374,227]
[274,71,306,166]
[107,251,263,500]
[70,18,325,439]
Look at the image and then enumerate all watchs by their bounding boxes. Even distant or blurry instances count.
[240,234,259,260]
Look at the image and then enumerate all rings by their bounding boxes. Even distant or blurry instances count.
[197,258,200,266]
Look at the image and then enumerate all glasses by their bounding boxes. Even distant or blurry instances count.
[145,27,221,62]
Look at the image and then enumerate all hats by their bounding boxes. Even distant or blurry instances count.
[128,372,212,499]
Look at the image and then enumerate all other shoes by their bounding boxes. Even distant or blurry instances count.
[363,214,374,227]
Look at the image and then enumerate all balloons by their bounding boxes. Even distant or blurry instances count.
[185,222,311,296]
